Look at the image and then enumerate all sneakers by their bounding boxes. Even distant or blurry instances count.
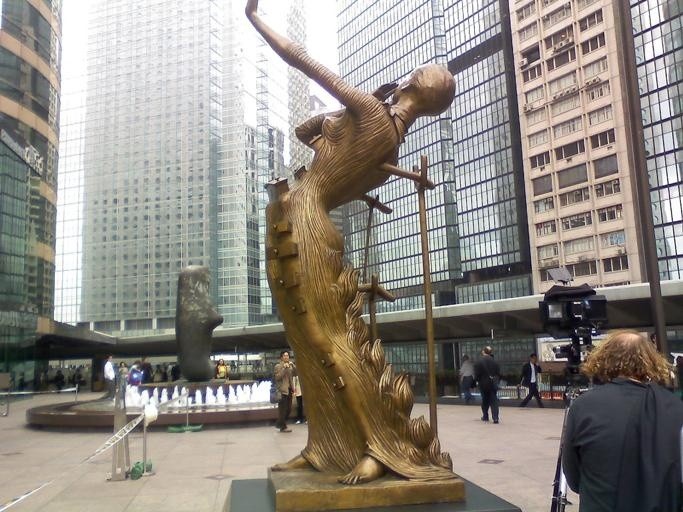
[481,416,498,423]
[275,425,292,432]
[295,419,308,425]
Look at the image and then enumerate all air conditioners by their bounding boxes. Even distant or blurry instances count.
[519,57,528,68]
[523,77,600,113]
[552,36,574,52]
[533,143,626,269]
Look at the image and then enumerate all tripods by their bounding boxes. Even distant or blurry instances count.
[551,384,588,512]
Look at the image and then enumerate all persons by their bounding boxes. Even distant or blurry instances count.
[459,346,500,423]
[9,353,236,399]
[517,353,544,407]
[245,0,455,485]
[273,351,308,432]
[561,329,683,512]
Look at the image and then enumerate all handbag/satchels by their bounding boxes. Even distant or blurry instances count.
[471,379,477,388]
[270,386,282,403]
